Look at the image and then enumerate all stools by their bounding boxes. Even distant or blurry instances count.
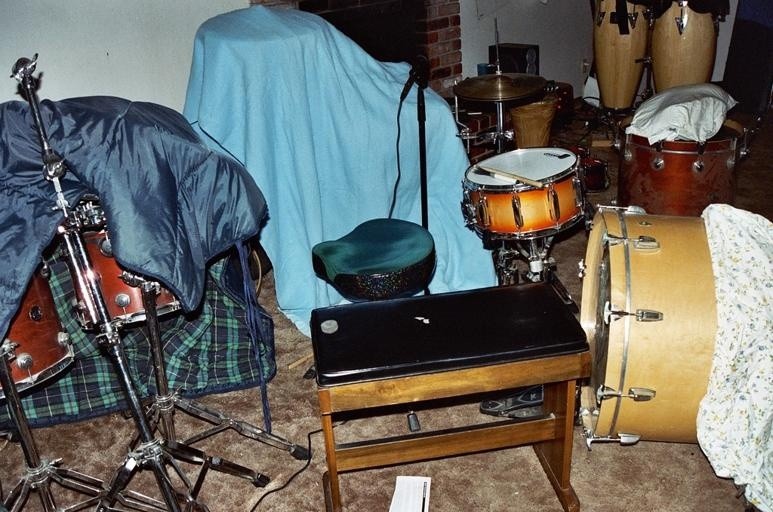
[305,218,437,432]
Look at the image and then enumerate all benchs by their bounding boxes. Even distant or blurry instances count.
[310,280,592,511]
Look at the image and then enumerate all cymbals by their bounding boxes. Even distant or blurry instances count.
[453,71,549,103]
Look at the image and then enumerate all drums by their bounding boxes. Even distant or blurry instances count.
[578,204,714,445]
[0,260,77,403]
[616,117,742,218]
[592,0,650,113]
[63,203,184,333]
[462,147,586,241]
[508,93,558,149]
[650,1,719,94]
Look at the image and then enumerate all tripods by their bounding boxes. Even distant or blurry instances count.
[0,53,312,512]
